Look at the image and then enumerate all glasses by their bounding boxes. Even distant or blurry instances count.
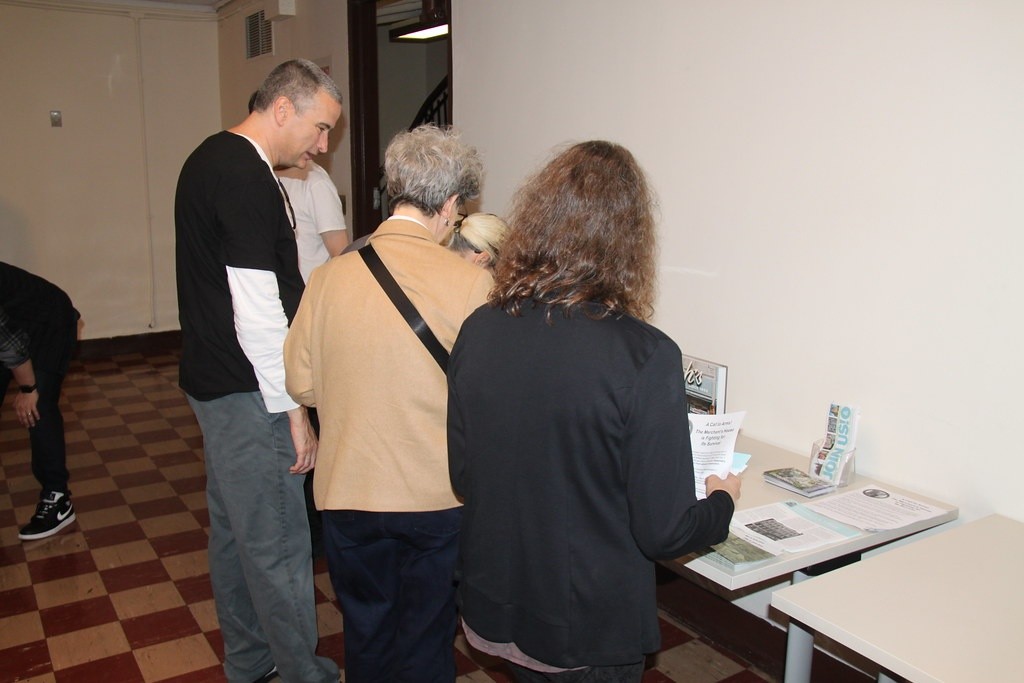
[454,195,467,225]
[278,177,296,229]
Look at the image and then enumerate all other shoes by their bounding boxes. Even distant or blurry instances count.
[254,665,278,683]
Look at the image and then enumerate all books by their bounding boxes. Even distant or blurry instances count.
[681,353,728,414]
[701,499,863,570]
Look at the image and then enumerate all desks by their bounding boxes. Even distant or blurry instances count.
[669,416,959,683]
[771,514,1024,683]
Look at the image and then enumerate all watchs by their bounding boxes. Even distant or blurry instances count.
[16,384,37,393]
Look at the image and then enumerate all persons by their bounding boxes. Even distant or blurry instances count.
[172,58,346,683]
[0,264,81,539]
[283,125,499,683]
[448,141,741,683]
[342,211,508,274]
[247,90,350,564]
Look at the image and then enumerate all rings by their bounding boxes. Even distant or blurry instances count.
[27,413,31,415]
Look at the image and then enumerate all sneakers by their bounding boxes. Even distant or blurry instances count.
[18,489,76,539]
